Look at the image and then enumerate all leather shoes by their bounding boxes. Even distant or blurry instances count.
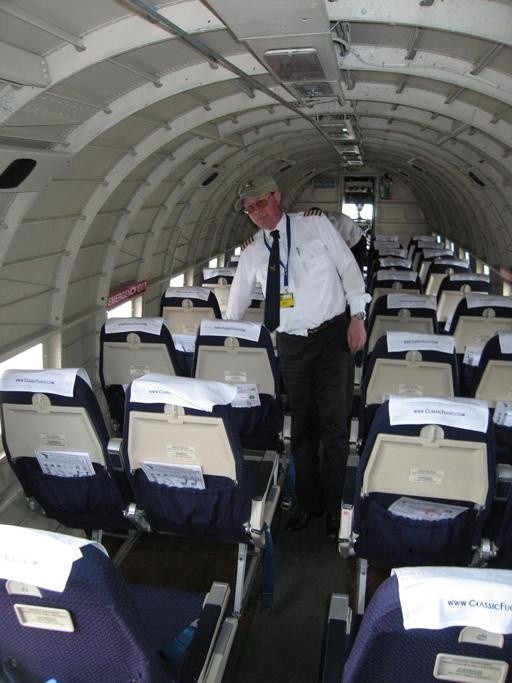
[286,510,308,532]
[326,514,340,537]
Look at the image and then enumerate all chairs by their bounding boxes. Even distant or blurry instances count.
[321,560,511,682]
[0,232,512,618]
[0,525,230,682]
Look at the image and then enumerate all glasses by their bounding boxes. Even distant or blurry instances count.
[242,198,268,214]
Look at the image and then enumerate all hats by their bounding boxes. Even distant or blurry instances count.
[234,175,278,211]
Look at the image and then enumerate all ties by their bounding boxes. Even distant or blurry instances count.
[264,230,280,331]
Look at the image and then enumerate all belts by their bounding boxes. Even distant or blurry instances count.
[308,314,345,336]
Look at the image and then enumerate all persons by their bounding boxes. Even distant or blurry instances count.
[309,207,367,277]
[223,171,373,540]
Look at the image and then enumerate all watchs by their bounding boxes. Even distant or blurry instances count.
[351,312,365,320]
